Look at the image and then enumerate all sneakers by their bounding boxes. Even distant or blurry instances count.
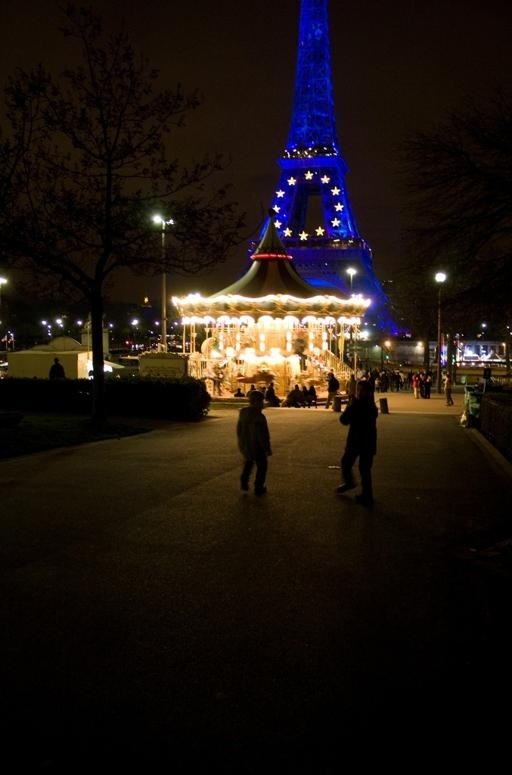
[240,474,267,496]
[335,482,372,500]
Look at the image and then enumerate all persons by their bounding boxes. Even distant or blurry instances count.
[284,384,301,407]
[356,357,434,399]
[266,382,282,406]
[150,340,182,352]
[261,386,266,393]
[233,387,245,397]
[235,391,274,497]
[246,384,257,397]
[325,372,340,409]
[307,384,317,408]
[442,374,454,407]
[333,380,380,501]
[47,357,65,380]
[297,386,309,407]
[346,374,356,403]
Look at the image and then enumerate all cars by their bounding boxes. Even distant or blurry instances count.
[116,357,141,376]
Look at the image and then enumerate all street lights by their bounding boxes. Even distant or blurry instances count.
[41,215,175,351]
[346,268,357,290]
[435,272,447,393]
[481,320,489,341]
